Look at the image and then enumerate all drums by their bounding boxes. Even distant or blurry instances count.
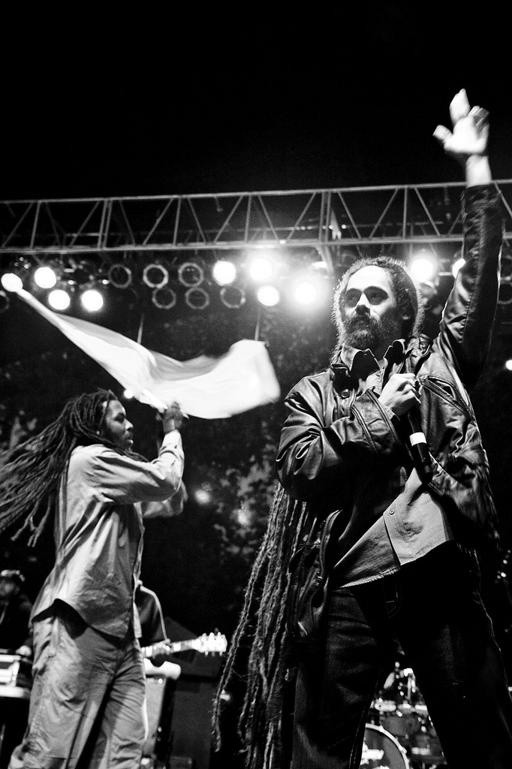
[373,697,428,745]
[358,719,413,769]
[405,716,447,766]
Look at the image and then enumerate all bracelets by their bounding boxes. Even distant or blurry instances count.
[162,420,181,432]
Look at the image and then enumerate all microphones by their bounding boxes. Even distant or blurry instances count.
[398,402,437,487]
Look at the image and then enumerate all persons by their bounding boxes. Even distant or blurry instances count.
[274,88,512,766]
[1,389,191,768]
[1,567,35,656]
[136,580,173,665]
